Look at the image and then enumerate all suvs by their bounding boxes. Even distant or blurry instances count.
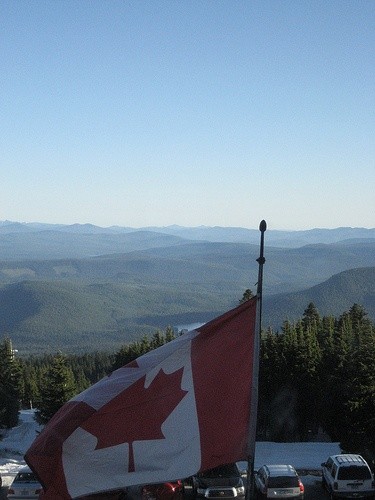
[320,454,375,500]
[193,458,245,500]
[142,480,184,500]
[252,462,304,500]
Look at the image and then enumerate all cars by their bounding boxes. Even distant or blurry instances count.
[6,465,43,500]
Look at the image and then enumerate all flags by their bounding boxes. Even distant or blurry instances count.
[23,290,259,500]
